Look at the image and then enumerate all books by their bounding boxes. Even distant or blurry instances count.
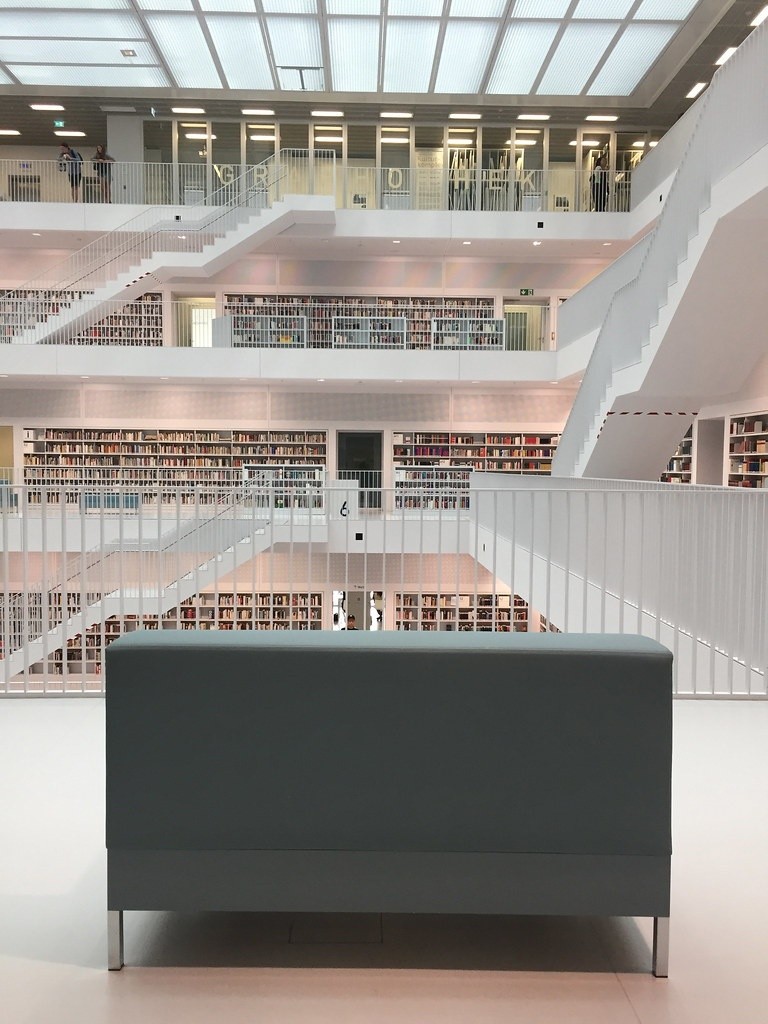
[539,614,563,633]
[224,296,308,349]
[378,298,499,351]
[126,594,217,631]
[159,431,326,508]
[728,417,768,488]
[311,298,377,350]
[65,292,162,346]
[22,429,157,504]
[219,595,321,630]
[393,433,485,509]
[396,594,528,632]
[660,425,692,484]
[0,593,120,675]
[487,435,559,470]
[0,289,80,344]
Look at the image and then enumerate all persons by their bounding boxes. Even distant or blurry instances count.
[588,156,609,212]
[341,614,359,630]
[56,143,81,202]
[90,144,115,203]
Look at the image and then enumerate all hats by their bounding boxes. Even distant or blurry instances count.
[347,614,354,621]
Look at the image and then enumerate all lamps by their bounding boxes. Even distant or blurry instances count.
[198,145,207,159]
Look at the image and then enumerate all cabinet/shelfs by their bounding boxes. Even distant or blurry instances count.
[394,591,529,632]
[21,426,329,514]
[0,288,164,347]
[447,148,525,210]
[0,592,323,675]
[211,289,506,350]
[583,150,644,212]
[657,424,693,484]
[727,411,768,489]
[393,431,562,510]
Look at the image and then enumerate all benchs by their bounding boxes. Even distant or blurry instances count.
[105,631,675,978]
[0,493,18,514]
[79,495,143,514]
